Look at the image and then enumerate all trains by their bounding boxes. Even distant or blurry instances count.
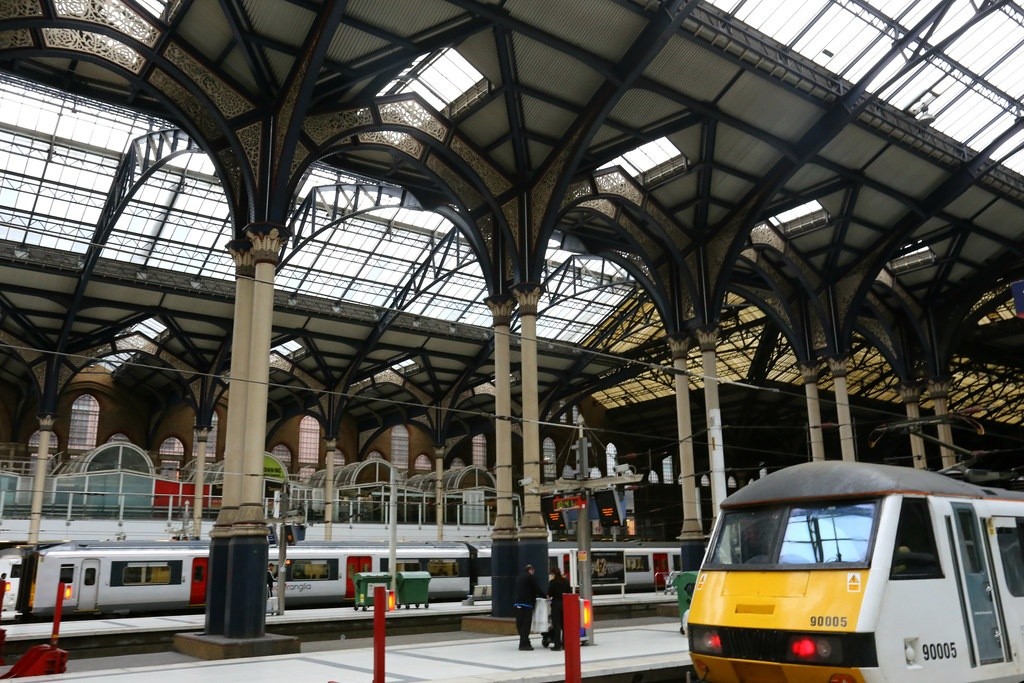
[0,514,711,653]
[682,459,1024,683]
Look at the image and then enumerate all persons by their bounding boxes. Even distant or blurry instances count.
[547,567,572,651]
[513,564,547,651]
[0,573,6,615]
[267,563,278,598]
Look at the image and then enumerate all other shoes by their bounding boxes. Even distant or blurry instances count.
[550,645,561,650]
[519,644,533,650]
